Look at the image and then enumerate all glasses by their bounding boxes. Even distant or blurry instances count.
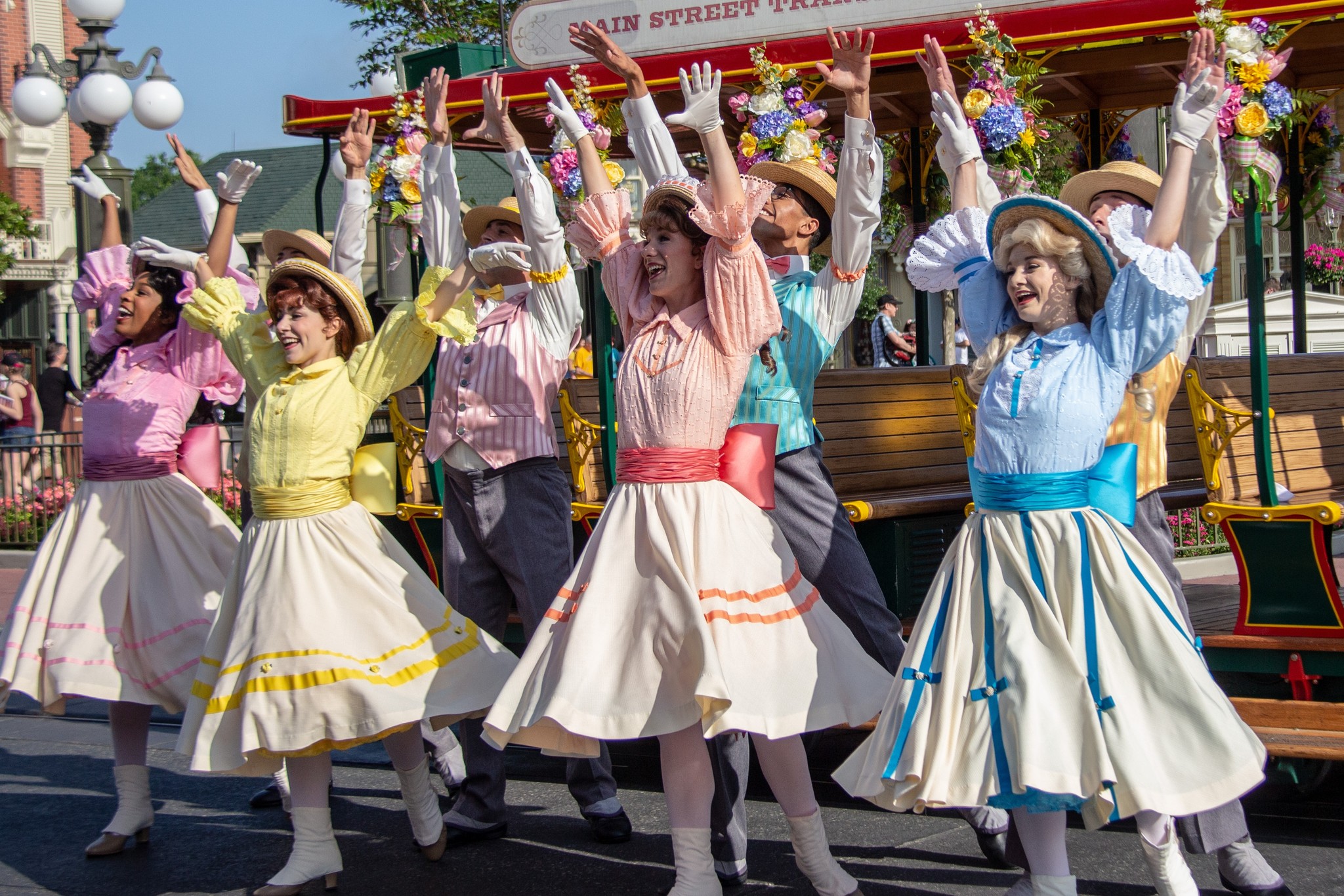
[771,186,818,237]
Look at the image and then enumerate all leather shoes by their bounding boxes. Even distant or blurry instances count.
[249,778,334,808]
[1216,835,1296,896]
[666,852,748,885]
[413,811,508,847]
[1003,871,1034,896]
[578,797,633,844]
[956,807,1021,869]
[432,743,466,803]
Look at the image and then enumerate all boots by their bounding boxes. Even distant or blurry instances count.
[786,801,864,896]
[1030,873,1078,896]
[85,765,155,855]
[666,827,722,896]
[273,760,296,830]
[1137,817,1201,896]
[252,806,345,896]
[391,752,448,860]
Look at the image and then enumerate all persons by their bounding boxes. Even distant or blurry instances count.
[915,26,1294,896]
[871,292,972,370]
[134,236,534,896]
[830,68,1270,896]
[480,65,900,896]
[0,352,44,500]
[544,20,1025,896]
[569,331,624,379]
[0,342,87,510]
[24,342,86,483]
[167,106,467,810]
[418,67,584,850]
[0,157,293,860]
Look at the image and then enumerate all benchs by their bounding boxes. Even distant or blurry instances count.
[947,364,1206,518]
[558,379,617,537]
[1184,352,1344,638]
[812,365,972,524]
[386,385,445,592]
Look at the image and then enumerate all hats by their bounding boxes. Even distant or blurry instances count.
[641,174,704,205]
[262,228,332,265]
[747,160,836,256]
[878,294,903,307]
[986,193,1119,311]
[267,258,376,359]
[463,197,522,245]
[1057,161,1164,219]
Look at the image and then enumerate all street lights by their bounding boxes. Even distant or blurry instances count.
[9,1,185,245]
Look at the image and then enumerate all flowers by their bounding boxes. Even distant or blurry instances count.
[538,63,627,223]
[726,43,839,175]
[365,83,429,221]
[961,3,1052,201]
[1194,0,1344,225]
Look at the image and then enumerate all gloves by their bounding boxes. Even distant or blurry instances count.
[136,235,210,274]
[544,77,596,147]
[664,60,724,135]
[215,158,263,205]
[467,242,532,275]
[930,89,983,166]
[1167,66,1232,156]
[67,163,122,209]
